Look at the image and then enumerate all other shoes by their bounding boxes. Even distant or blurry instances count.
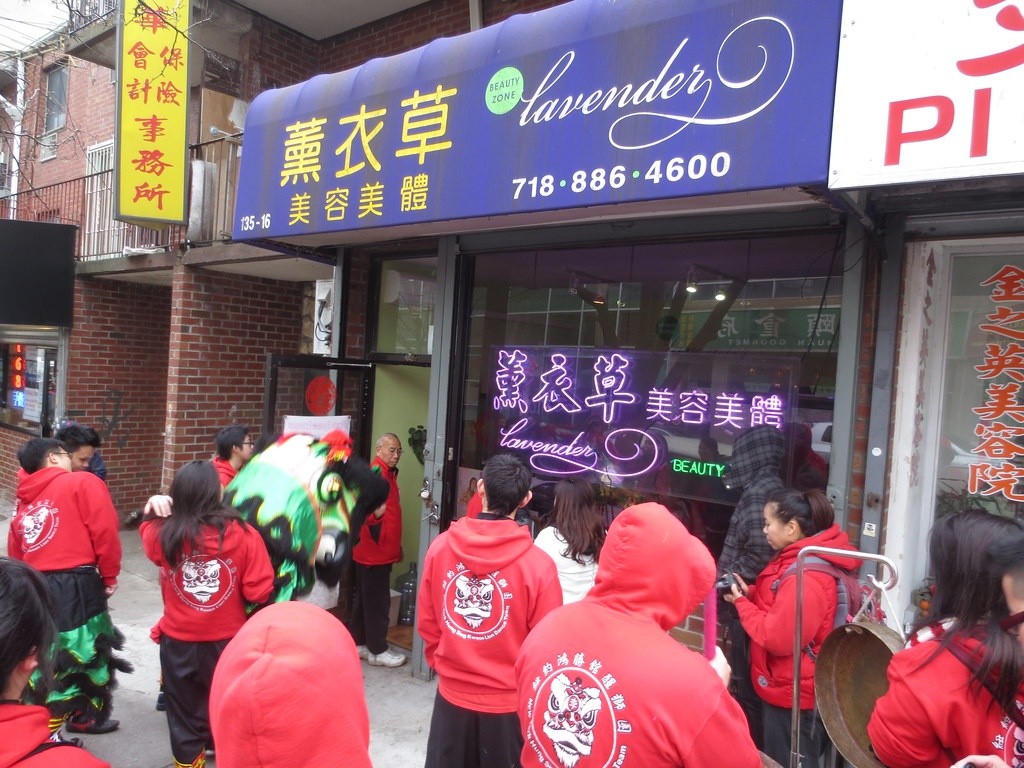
[156,683,167,711]
[50,729,83,749]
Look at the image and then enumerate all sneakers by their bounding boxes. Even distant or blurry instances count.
[65,710,119,733]
[367,648,407,668]
[357,645,369,659]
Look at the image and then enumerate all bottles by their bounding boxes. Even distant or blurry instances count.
[396,562,417,625]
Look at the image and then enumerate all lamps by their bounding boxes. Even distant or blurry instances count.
[686,270,700,292]
[715,275,728,302]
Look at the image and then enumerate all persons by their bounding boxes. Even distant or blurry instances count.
[416,454,563,768]
[462,390,837,561]
[0,554,114,768]
[346,433,408,668]
[139,460,276,767]
[292,452,390,613]
[513,502,768,768]
[709,424,792,754]
[212,426,255,492]
[209,600,375,768]
[52,423,107,481]
[947,535,1024,768]
[721,486,863,768]
[867,509,1024,768]
[11,436,123,735]
[532,478,610,606]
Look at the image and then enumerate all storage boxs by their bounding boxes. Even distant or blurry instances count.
[387,588,402,628]
[0,408,22,424]
[18,421,28,428]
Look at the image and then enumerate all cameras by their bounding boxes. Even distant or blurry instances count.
[714,572,743,595]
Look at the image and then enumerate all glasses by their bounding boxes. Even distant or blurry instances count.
[48,452,72,459]
[238,442,254,447]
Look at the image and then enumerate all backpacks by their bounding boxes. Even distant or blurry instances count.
[769,557,887,661]
